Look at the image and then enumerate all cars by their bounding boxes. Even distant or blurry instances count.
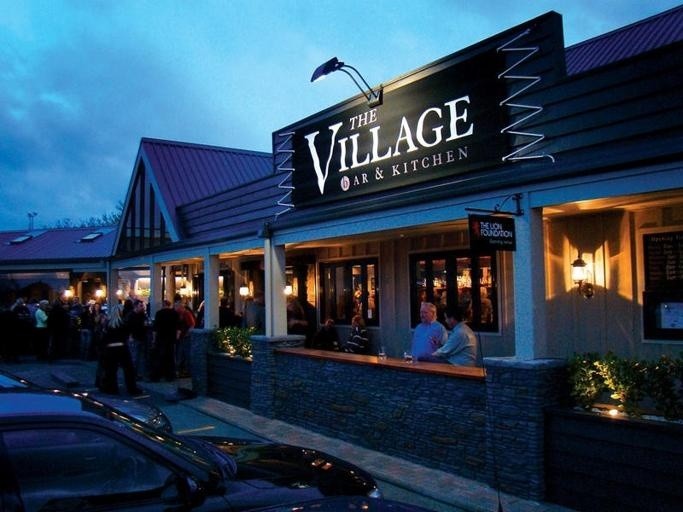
[249,494,428,512]
[0,388,387,512]
[0,370,172,452]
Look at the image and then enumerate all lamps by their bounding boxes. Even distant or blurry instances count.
[310,56,384,109]
[571,249,593,299]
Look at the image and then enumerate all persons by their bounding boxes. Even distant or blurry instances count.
[410,302,448,364]
[340,314,369,354]
[431,304,478,368]
[352,286,494,325]
[310,318,342,352]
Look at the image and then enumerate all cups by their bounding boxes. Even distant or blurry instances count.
[403,351,413,364]
[377,346,388,361]
